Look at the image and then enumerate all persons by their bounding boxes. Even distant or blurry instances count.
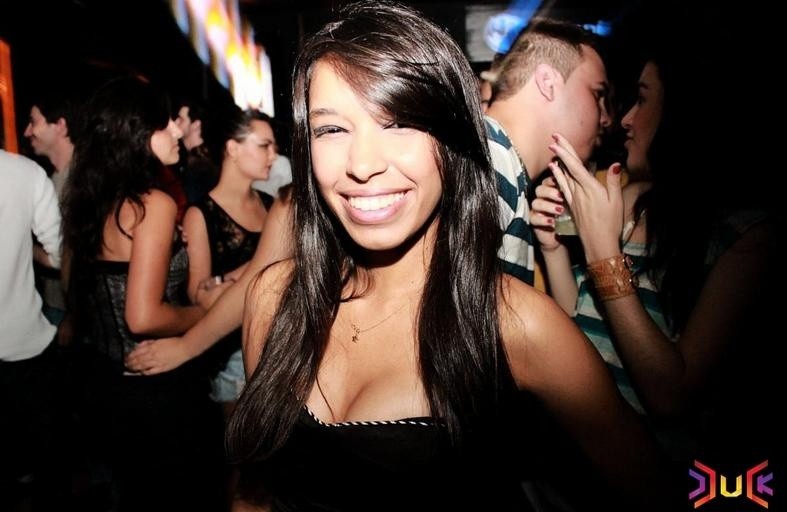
[1,0,787,511]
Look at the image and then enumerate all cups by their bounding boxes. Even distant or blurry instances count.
[551,158,599,238]
